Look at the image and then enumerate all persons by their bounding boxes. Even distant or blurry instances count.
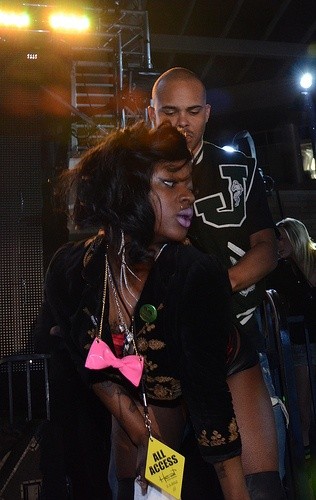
[276,217,315,439]
[27,117,248,499]
[147,67,280,439]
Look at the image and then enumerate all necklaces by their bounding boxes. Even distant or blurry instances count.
[85,232,167,387]
